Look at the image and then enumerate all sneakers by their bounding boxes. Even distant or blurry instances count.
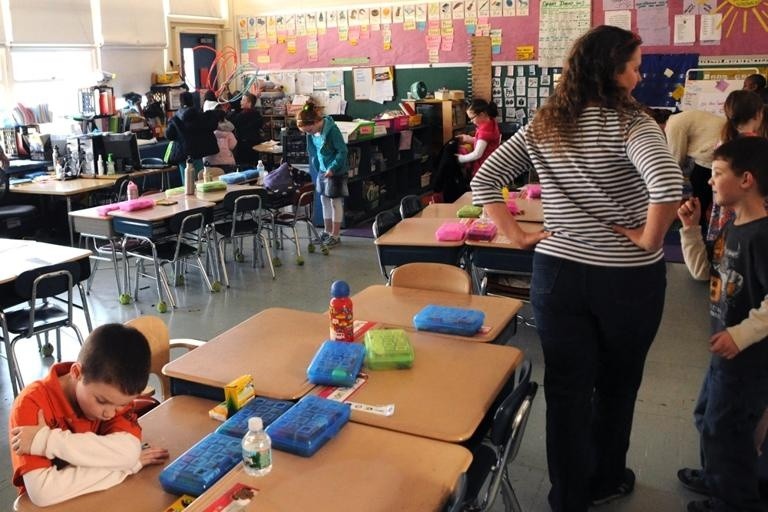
[592,468,635,505]
[676,467,709,493]
[324,235,341,248]
[687,496,722,511]
[313,231,329,244]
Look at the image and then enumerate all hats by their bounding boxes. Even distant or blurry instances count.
[180,92,193,105]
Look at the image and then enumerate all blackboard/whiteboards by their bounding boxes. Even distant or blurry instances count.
[242,61,564,130]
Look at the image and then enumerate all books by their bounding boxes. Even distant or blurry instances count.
[399,102,414,115]
[90,89,123,132]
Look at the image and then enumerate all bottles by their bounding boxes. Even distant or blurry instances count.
[51,144,104,180]
[329,279,355,343]
[183,155,196,196]
[239,415,273,478]
[257,160,267,172]
[125,181,138,201]
[202,160,212,182]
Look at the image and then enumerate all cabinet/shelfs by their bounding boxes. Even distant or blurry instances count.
[281,114,438,228]
[401,97,468,147]
[261,113,296,166]
[150,84,189,126]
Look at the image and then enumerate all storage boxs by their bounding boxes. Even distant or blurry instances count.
[357,122,375,141]
[371,115,409,134]
[405,113,423,128]
[284,103,325,117]
[157,71,178,84]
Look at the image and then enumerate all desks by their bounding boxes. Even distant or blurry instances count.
[137,141,181,187]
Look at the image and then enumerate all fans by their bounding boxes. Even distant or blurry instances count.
[409,80,427,99]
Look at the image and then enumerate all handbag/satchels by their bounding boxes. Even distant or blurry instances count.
[444,171,468,202]
[164,141,187,165]
[429,154,454,190]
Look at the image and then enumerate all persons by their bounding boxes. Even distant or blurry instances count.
[8,323,170,506]
[704,91,763,242]
[640,105,729,241]
[741,74,767,123]
[467,24,685,512]
[663,138,768,510]
[203,89,220,112]
[453,98,501,180]
[162,90,348,250]
